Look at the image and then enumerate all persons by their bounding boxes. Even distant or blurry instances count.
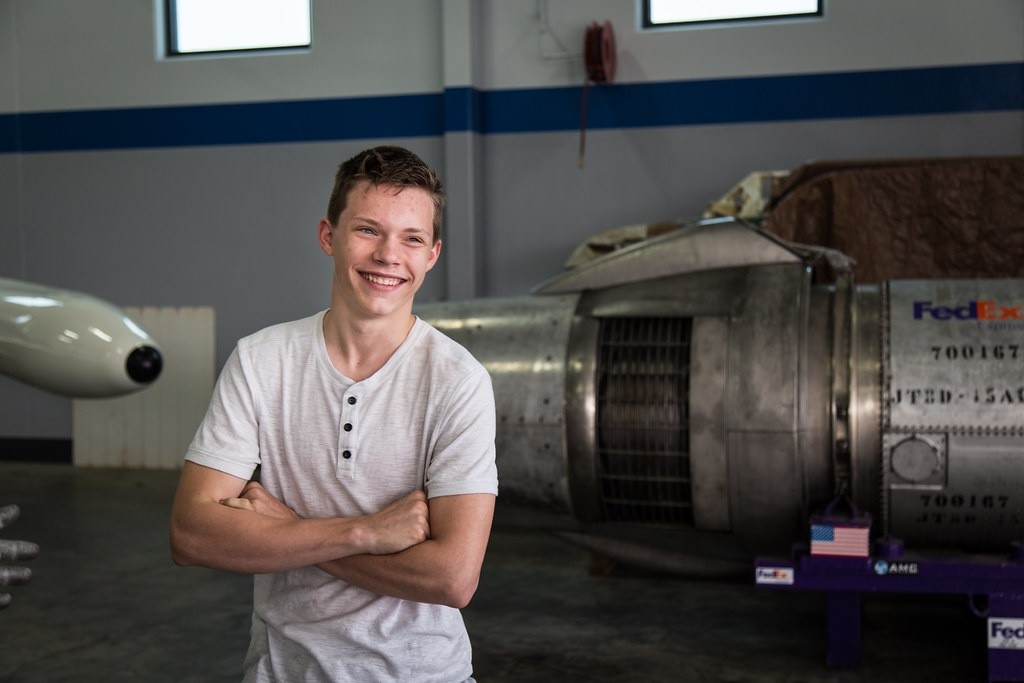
[170,146,499,683]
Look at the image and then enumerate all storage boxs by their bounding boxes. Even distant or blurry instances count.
[808,512,872,559]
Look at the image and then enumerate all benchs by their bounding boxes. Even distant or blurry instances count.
[757,552,1024,683]
